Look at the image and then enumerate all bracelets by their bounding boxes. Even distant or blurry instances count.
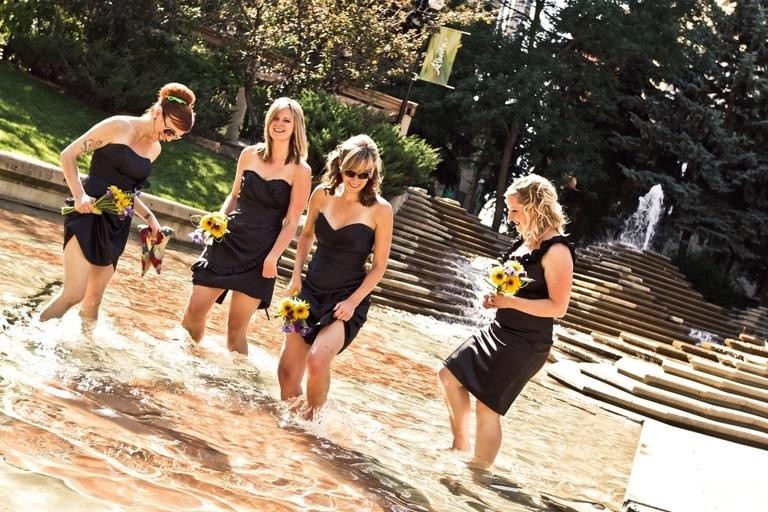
[143,212,153,220]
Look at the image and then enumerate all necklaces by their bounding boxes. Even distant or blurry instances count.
[522,226,551,246]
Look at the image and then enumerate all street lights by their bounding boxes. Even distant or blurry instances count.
[394,0,448,133]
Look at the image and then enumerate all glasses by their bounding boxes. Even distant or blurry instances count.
[340,170,370,179]
[163,111,183,140]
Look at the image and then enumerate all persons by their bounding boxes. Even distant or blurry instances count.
[437,172,576,467]
[179,94,313,355]
[38,80,198,324]
[277,131,394,422]
